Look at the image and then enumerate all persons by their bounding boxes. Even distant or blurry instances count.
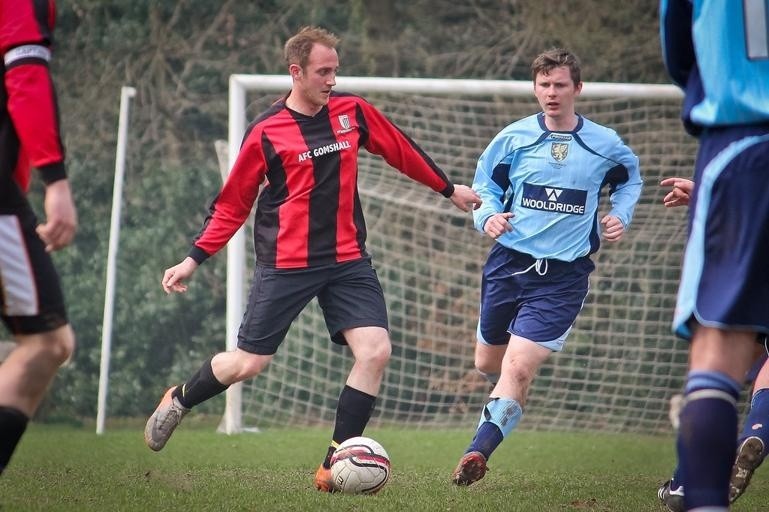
[648,1,768,510]
[0,2,79,473]
[450,46,646,488]
[140,22,483,498]
[653,174,769,510]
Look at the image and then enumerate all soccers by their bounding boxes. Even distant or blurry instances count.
[330,435,391,494]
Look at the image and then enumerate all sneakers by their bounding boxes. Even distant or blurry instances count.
[657,478,687,512]
[451,451,488,489]
[728,435,765,504]
[315,463,334,492]
[144,381,192,452]
